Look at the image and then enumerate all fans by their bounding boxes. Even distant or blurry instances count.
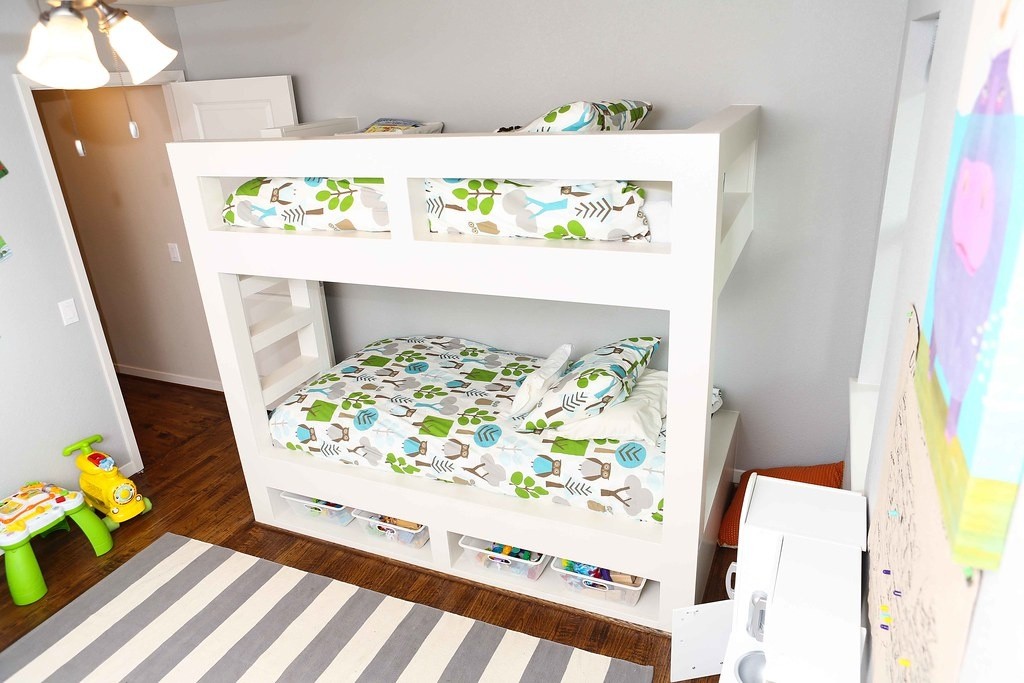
[16,0,181,155]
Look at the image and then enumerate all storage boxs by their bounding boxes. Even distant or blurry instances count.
[280,492,356,527]
[457,534,553,580]
[550,557,649,606]
[351,508,429,549]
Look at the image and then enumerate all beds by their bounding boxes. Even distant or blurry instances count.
[165,102,763,644]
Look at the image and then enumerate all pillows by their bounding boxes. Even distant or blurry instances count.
[716,459,846,548]
[519,99,654,132]
[517,336,663,433]
[562,366,668,444]
[510,342,574,418]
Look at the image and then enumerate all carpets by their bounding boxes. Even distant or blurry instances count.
[0,533,654,683]
